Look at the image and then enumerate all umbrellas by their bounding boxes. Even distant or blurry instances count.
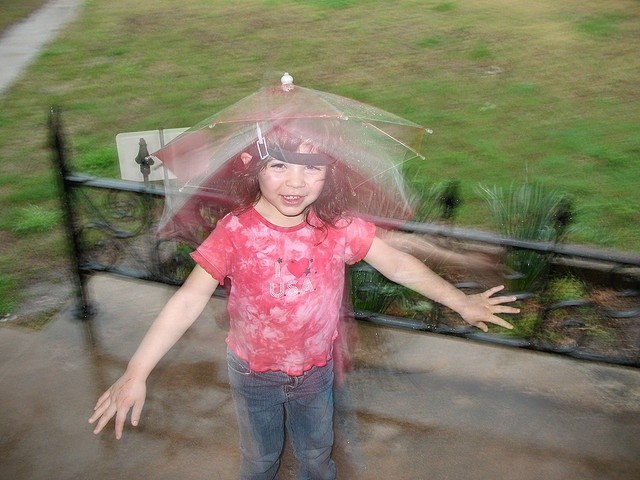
[152,72,431,251]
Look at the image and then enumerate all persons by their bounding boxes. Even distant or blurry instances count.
[89,97,520,480]
